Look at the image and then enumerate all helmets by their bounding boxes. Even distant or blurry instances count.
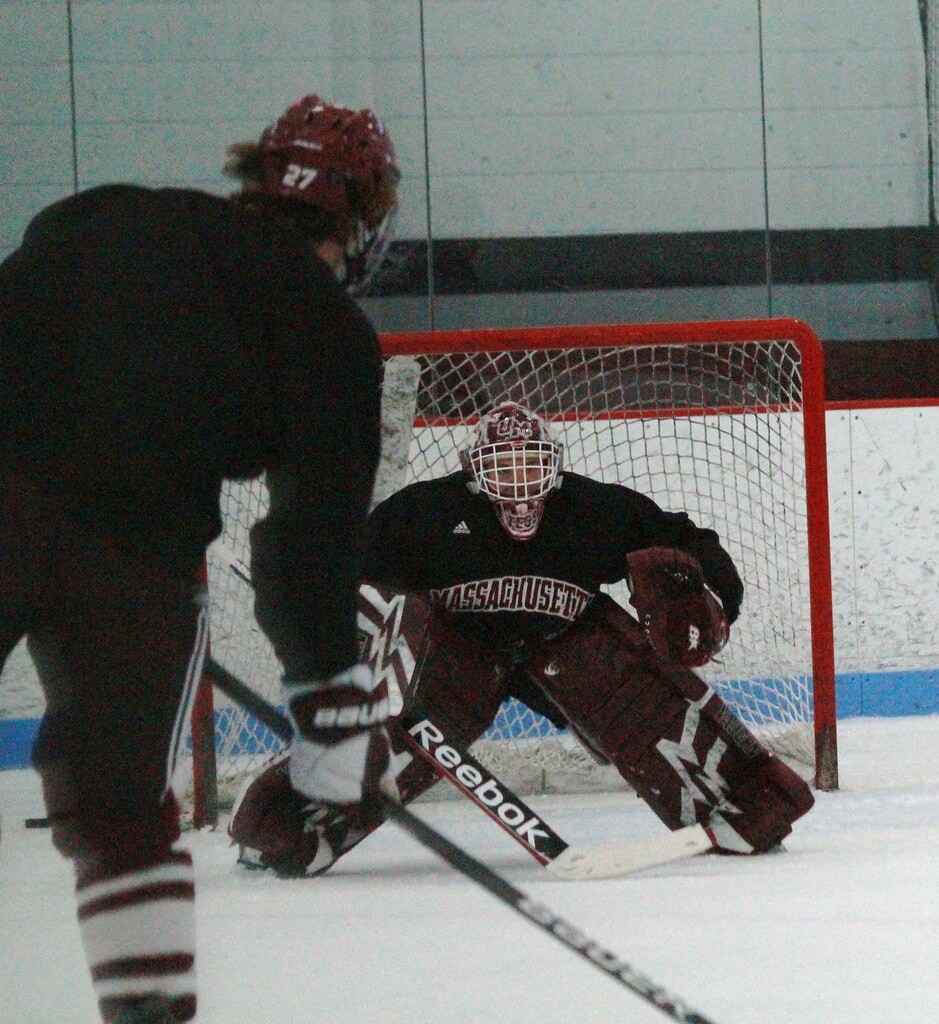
[458,402,563,541]
[233,94,401,296]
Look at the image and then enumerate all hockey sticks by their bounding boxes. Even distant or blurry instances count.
[203,657,719,1023]
[388,705,719,879]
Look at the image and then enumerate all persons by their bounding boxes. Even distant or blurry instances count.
[0,95,401,1024]
[227,404,816,879]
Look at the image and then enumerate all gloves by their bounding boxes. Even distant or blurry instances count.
[281,663,399,804]
